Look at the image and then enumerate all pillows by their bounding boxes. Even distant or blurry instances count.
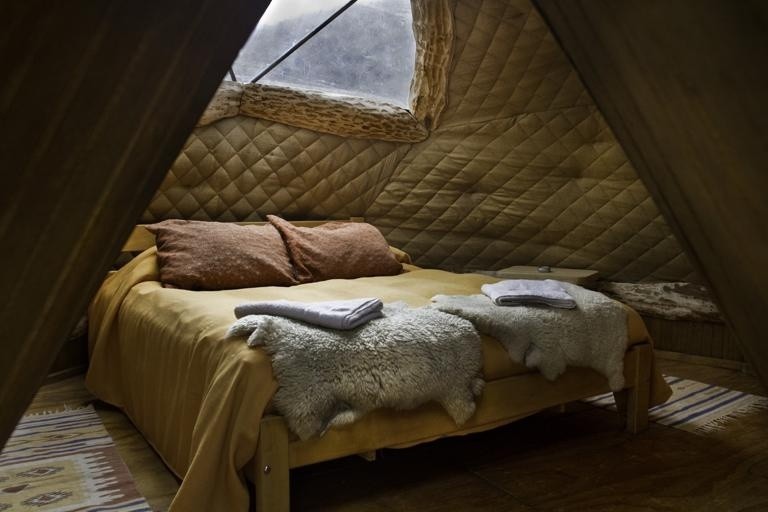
[142,218,299,291]
[264,211,403,283]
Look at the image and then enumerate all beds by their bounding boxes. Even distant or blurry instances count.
[87,217,673,511]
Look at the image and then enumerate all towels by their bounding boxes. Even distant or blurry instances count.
[480,275,578,309]
[235,296,384,332]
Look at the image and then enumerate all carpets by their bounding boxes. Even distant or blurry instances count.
[0,405,150,512]
[581,371,767,440]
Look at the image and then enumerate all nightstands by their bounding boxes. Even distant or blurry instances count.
[493,264,598,291]
